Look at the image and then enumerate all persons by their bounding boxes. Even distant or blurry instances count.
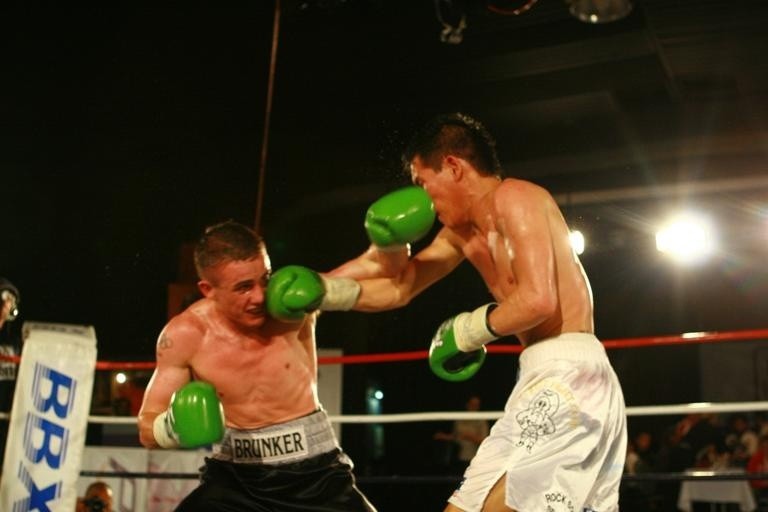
[436,397,488,471]
[620,417,768,511]
[76,482,114,512]
[269,111,629,511]
[103,376,150,446]
[1,284,18,326]
[137,183,436,512]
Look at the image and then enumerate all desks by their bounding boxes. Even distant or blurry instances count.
[677,466,758,512]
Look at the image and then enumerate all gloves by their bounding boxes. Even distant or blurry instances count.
[266,264,363,320]
[428,302,503,383]
[153,380,226,451]
[362,186,436,256]
